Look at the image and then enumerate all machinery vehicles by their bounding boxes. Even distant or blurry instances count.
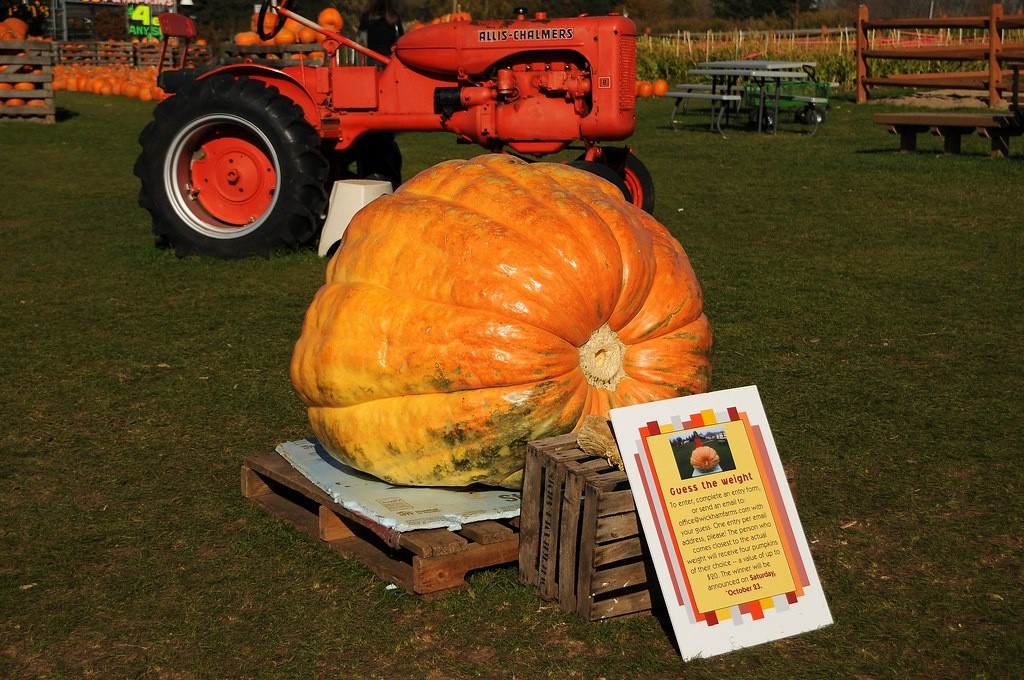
[131,0,655,262]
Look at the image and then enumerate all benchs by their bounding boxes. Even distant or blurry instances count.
[874,61,1023,158]
[663,60,829,138]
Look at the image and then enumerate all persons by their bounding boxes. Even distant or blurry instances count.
[356,1,404,72]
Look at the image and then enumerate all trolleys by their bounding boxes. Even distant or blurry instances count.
[743,64,830,130]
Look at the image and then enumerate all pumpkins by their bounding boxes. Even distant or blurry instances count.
[291,153,712,490]
[0,6,669,116]
